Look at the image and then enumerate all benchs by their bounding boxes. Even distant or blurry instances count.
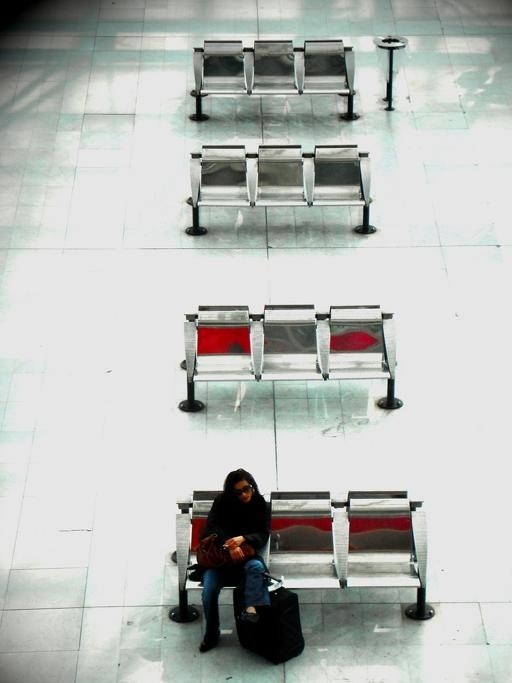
[179,304,404,412]
[185,144,377,236]
[189,40,360,121]
[167,490,435,622]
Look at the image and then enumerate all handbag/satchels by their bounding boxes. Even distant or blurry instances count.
[197,533,255,567]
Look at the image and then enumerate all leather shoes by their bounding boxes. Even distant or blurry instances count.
[237,611,259,622]
[199,636,219,652]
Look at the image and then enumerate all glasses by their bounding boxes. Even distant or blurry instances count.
[233,484,252,496]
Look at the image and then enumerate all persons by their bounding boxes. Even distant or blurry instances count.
[199,469,271,652]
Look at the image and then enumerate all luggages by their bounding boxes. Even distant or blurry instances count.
[233,573,304,665]
